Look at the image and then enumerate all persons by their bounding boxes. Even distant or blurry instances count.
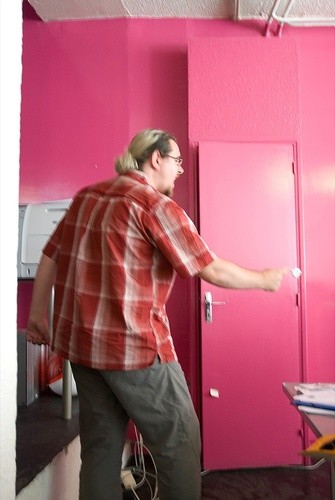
[23,129,291,500]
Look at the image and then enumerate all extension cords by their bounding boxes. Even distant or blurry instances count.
[120,469,136,491]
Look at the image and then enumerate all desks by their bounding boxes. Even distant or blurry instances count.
[281,382,335,500]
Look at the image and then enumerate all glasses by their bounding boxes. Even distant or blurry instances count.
[165,155,183,166]
